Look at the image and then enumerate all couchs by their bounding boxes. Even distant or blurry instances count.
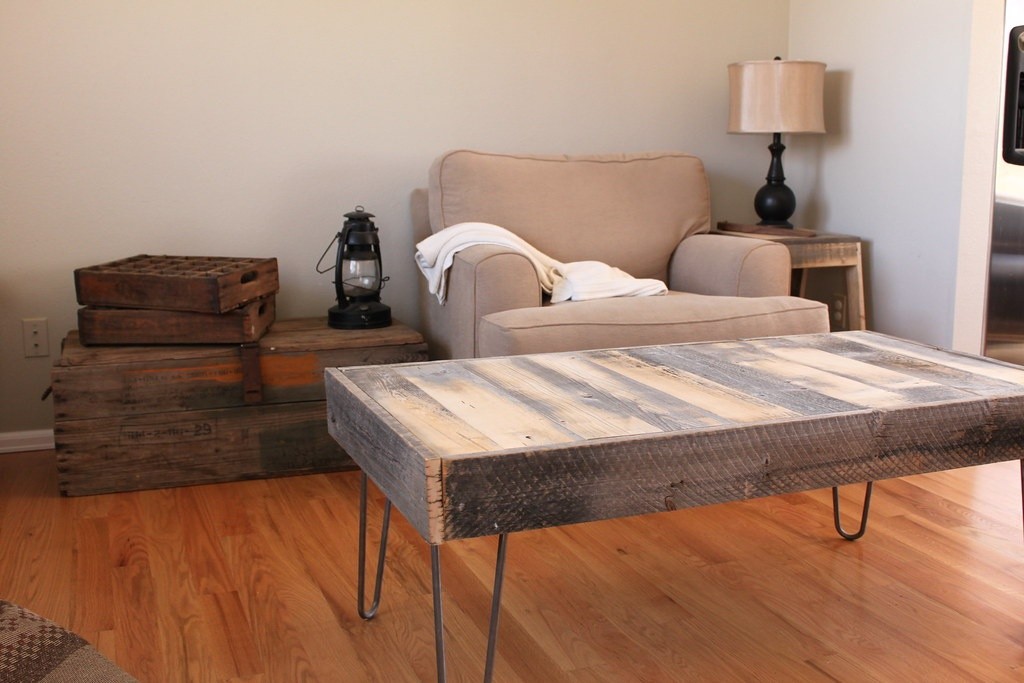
[421,146,830,358]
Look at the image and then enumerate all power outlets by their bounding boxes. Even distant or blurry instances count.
[22,317,51,358]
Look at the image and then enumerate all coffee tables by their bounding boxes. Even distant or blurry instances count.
[323,329,1024,683]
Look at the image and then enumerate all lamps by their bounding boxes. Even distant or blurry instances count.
[315,203,395,330]
[727,54,828,227]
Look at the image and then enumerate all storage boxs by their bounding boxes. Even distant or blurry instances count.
[74,254,280,314]
[42,315,429,498]
[78,295,277,348]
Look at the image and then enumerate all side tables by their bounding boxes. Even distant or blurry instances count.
[711,229,867,331]
